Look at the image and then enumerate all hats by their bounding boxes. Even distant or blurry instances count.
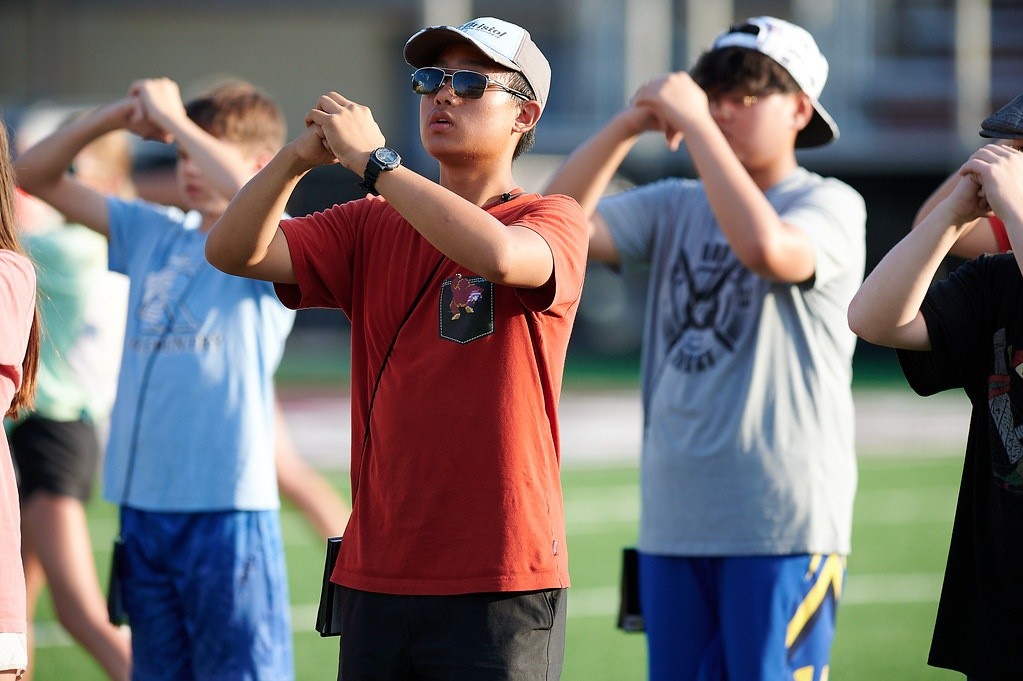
[403,17,552,121]
[710,16,838,150]
[979,93,1023,140]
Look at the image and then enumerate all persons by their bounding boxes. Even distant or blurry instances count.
[847,95,1023,681]
[538,15,867,681]
[0,77,297,681]
[204,17,590,681]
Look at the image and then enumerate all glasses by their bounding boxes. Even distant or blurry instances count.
[410,66,529,101]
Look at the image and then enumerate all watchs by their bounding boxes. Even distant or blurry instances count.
[357,147,405,198]
